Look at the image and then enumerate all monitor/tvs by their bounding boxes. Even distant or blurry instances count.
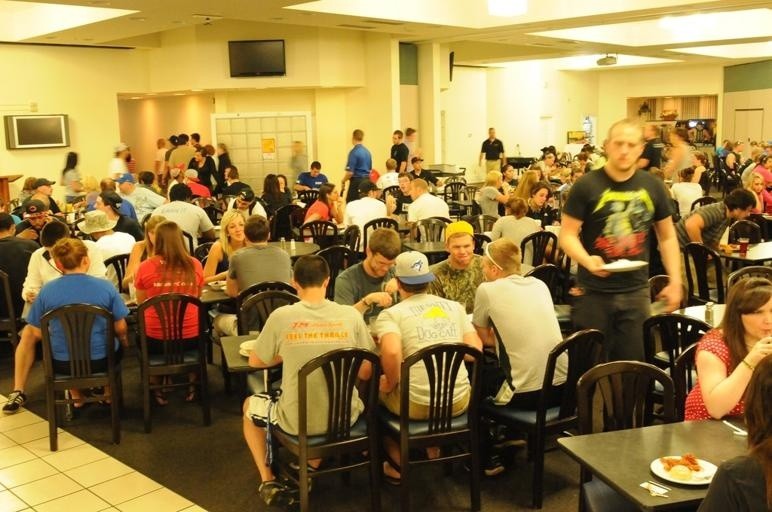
[228,40,286,77]
[4,114,70,149]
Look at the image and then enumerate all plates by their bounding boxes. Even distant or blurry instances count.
[602,259,648,272]
[238,349,256,356]
[650,452,718,484]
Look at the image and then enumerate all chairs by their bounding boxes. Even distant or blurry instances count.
[473,232,491,255]
[690,198,719,212]
[298,190,322,205]
[729,221,762,246]
[670,341,700,424]
[576,361,677,512]
[646,276,689,311]
[466,185,480,200]
[480,329,606,508]
[182,230,196,261]
[745,214,772,237]
[382,185,400,198]
[299,221,339,243]
[341,225,362,252]
[137,294,212,432]
[271,347,380,512]
[410,217,453,243]
[727,266,772,299]
[363,219,399,263]
[270,205,303,243]
[521,233,558,267]
[237,292,301,335]
[201,206,225,225]
[447,180,467,204]
[683,243,725,305]
[1,270,25,357]
[526,264,562,306]
[315,245,356,301]
[209,282,299,323]
[380,343,483,512]
[714,239,772,270]
[41,303,123,453]
[104,252,130,290]
[197,242,229,274]
[406,241,449,263]
[643,314,714,426]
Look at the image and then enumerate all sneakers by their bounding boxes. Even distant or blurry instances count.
[258,478,291,505]
[464,452,504,475]
[288,459,317,474]
[495,432,526,451]
[2,391,27,413]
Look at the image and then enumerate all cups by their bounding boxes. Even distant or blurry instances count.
[66,213,75,224]
[128,282,137,302]
[737,237,749,254]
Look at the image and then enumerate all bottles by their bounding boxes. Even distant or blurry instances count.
[290,239,295,250]
[704,300,714,324]
[281,236,286,252]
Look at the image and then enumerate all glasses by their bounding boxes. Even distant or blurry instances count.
[485,241,502,270]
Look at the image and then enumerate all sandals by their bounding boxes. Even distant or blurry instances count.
[185,382,200,401]
[149,385,168,405]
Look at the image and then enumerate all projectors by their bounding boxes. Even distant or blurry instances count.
[598,58,617,65]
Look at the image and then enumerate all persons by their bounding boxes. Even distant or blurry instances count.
[558,117,682,429]
[110,143,129,181]
[21,178,61,214]
[403,127,423,172]
[213,165,252,195]
[636,122,718,219]
[260,173,292,234]
[478,170,509,218]
[121,215,168,290]
[390,129,409,174]
[289,140,308,172]
[524,144,606,179]
[113,173,146,223]
[152,183,215,254]
[409,157,443,188]
[2,220,98,415]
[406,178,450,242]
[334,227,403,326]
[202,208,250,284]
[92,190,145,242]
[471,239,569,479]
[242,254,374,509]
[715,139,772,214]
[24,237,131,410]
[154,131,232,198]
[294,161,329,200]
[426,221,489,315]
[86,178,137,221]
[657,188,757,295]
[684,277,772,421]
[0,212,41,338]
[344,180,387,264]
[227,187,268,220]
[695,355,772,512]
[501,165,517,195]
[277,173,292,202]
[77,210,137,293]
[14,200,59,247]
[135,171,168,208]
[60,151,85,193]
[386,172,414,216]
[478,127,506,176]
[376,158,399,190]
[133,220,205,407]
[342,129,372,204]
[303,183,344,227]
[373,250,483,489]
[212,213,295,341]
[492,196,544,266]
[525,182,561,230]
[514,169,539,198]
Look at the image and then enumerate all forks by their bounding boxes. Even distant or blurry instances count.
[644,484,669,498]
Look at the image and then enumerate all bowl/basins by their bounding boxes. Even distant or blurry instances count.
[208,280,225,291]
[240,338,269,354]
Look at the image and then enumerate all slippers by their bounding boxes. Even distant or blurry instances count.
[383,456,404,486]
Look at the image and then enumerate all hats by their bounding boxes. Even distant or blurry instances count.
[184,169,200,182]
[411,157,424,163]
[171,168,180,177]
[33,178,56,188]
[239,188,254,201]
[394,251,433,284]
[23,199,47,218]
[114,144,129,152]
[99,190,127,216]
[358,181,382,196]
[446,221,475,244]
[168,136,178,143]
[77,210,117,234]
[117,173,135,182]
